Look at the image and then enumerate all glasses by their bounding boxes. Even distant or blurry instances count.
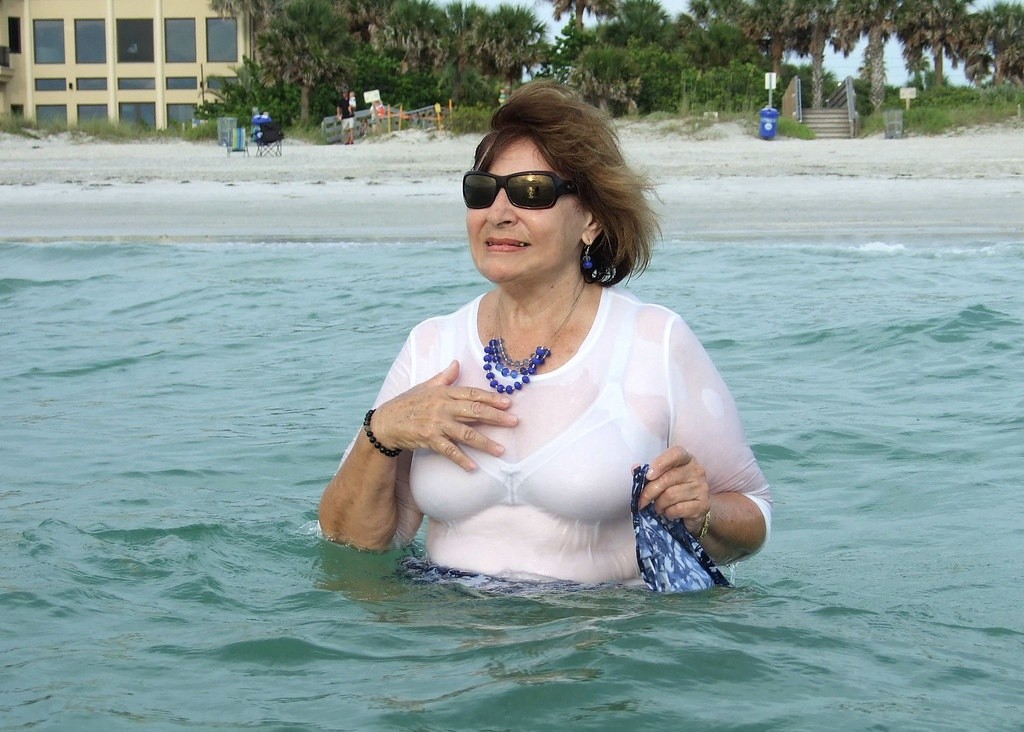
[462,170,577,210]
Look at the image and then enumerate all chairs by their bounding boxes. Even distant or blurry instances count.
[254,120,285,157]
[223,128,249,158]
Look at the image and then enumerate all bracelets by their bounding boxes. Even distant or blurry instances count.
[697,510,710,544]
[363,409,402,457]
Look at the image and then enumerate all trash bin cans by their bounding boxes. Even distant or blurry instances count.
[760,109,778,139]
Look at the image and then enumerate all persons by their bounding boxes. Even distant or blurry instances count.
[318,82,773,593]
[359,117,369,136]
[370,100,383,132]
[535,186,539,196]
[337,90,356,145]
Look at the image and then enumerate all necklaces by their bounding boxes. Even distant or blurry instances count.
[483,281,585,394]
